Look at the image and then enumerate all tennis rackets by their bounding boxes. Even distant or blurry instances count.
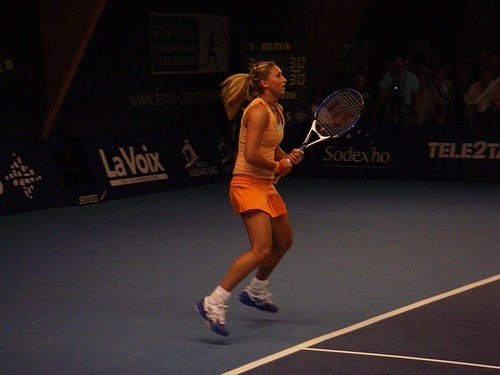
[274,88,364,183]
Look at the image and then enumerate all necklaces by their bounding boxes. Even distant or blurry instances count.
[260,96,277,111]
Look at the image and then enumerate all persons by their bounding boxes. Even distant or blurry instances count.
[313,48,500,124]
[197,61,304,337]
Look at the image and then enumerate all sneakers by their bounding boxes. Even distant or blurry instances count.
[239,285,278,313]
[197,294,231,338]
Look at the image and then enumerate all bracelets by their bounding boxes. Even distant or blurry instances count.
[273,161,284,174]
